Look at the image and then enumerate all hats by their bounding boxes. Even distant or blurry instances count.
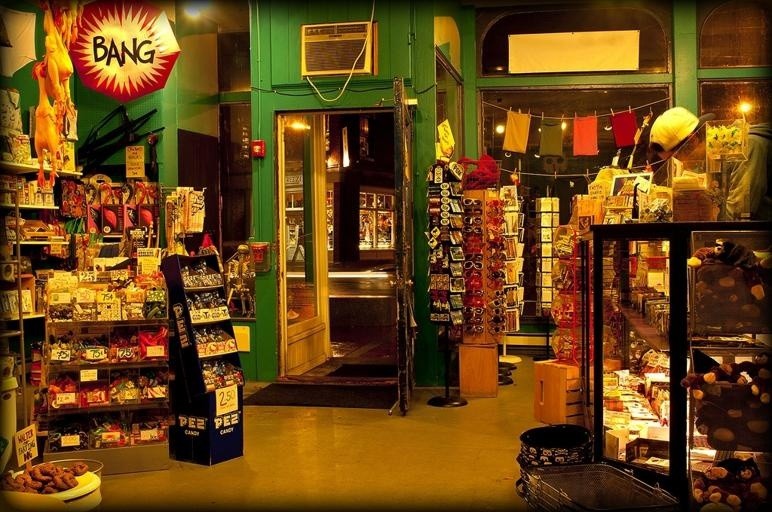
[650,106,716,153]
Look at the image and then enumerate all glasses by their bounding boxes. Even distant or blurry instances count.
[462,198,508,335]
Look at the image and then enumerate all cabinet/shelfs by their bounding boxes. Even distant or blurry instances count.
[581,222,772,512]
[0,161,84,438]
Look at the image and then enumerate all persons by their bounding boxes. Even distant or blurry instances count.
[650,105,772,223]
